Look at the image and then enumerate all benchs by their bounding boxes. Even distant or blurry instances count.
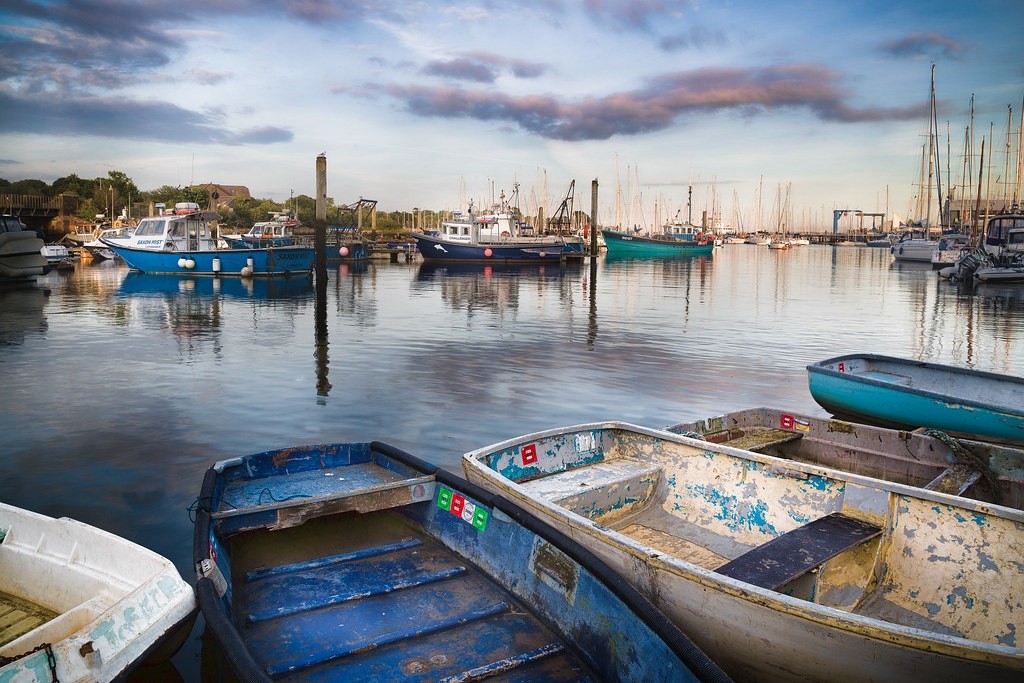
[854,371,911,384]
[922,469,981,495]
[715,427,802,452]
[518,457,662,522]
[707,513,885,590]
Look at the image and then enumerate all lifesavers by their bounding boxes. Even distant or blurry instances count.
[500,231,510,236]
[176,209,208,215]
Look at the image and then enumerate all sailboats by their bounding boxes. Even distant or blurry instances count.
[714,174,809,249]
[891,63,1024,282]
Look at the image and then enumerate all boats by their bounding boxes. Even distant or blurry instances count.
[408,180,587,265]
[0,354,1024,683]
[83,199,378,273]
[600,230,714,253]
[0,213,76,279]
[866,240,890,247]
